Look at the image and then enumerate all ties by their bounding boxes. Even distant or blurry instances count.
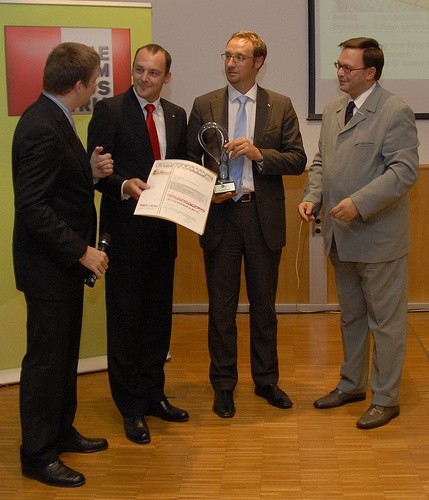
[229,95,249,202]
[144,104,161,160]
[345,101,356,124]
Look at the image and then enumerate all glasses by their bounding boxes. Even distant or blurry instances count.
[334,61,368,74]
[221,53,255,63]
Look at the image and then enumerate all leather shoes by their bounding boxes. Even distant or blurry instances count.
[21,460,86,487]
[255,384,293,407]
[313,387,366,409]
[145,399,190,422]
[212,390,235,417]
[60,431,108,453]
[123,415,151,443]
[356,404,399,429]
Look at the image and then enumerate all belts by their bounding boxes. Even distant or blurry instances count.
[228,193,257,202]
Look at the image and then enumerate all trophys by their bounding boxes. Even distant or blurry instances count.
[198,121,237,195]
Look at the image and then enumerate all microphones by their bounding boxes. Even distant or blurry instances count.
[85,232,111,287]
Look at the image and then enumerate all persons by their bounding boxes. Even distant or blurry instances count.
[297,37,420,429]
[187,32,308,418]
[12,42,114,488]
[85,43,188,443]
